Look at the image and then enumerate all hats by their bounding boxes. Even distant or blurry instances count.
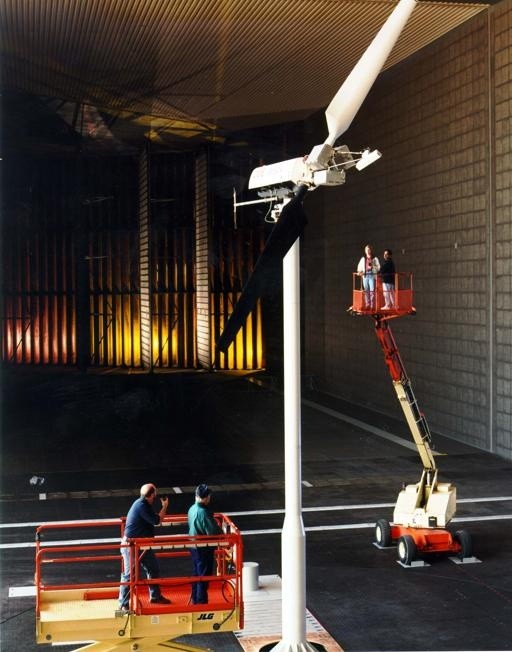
[195,484,210,496]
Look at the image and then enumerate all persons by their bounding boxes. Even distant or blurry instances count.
[188,482,221,604]
[357,245,396,311]
[118,482,171,615]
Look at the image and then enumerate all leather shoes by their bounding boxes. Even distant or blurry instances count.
[120,605,129,610]
[149,594,171,604]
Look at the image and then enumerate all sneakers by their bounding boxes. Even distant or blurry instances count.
[363,306,396,310]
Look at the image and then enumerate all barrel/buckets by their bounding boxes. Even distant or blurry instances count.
[242,562,259,591]
